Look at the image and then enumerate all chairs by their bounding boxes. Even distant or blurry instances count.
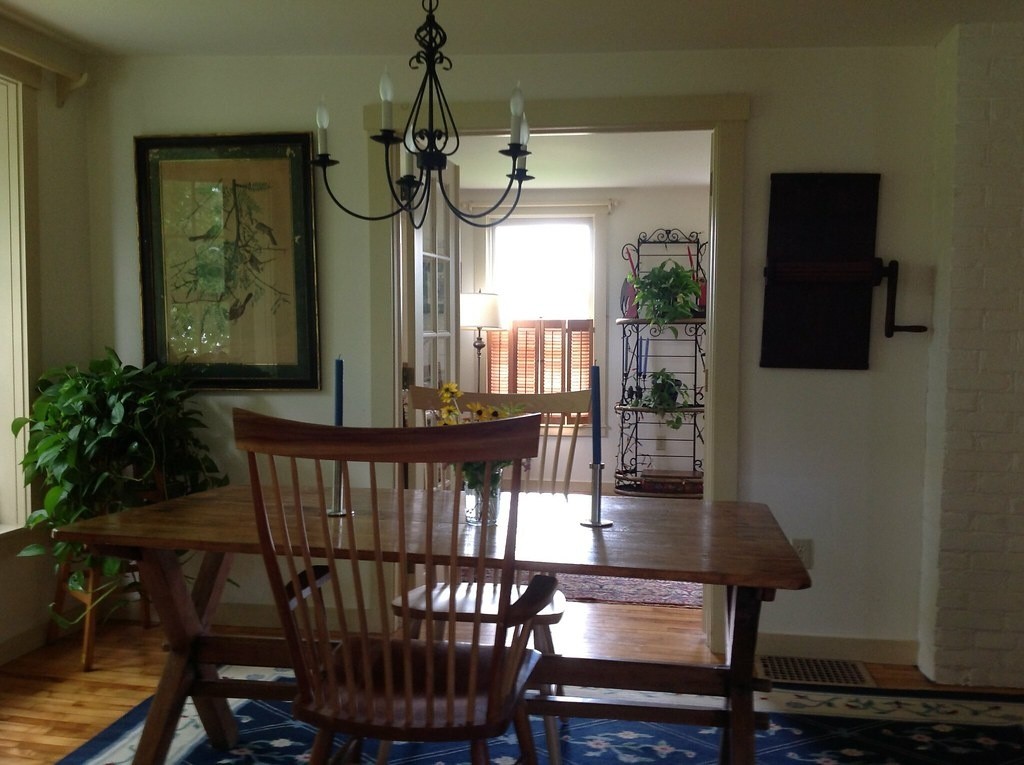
[232,405,558,765]
[392,388,592,765]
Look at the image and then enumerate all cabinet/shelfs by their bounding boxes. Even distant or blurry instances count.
[615,228,706,499]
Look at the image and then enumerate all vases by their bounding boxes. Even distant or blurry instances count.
[472,466,502,526]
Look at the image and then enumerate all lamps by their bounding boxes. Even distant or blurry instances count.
[309,1,535,231]
[460,291,506,393]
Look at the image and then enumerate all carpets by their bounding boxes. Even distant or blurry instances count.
[54,656,1024,764]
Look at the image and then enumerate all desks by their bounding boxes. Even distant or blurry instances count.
[50,481,812,765]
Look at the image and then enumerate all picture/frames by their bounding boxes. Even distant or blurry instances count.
[131,131,323,393]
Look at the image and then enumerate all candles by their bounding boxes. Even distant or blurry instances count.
[335,360,343,426]
[592,365,601,463]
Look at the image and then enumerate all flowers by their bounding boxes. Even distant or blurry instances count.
[436,384,532,518]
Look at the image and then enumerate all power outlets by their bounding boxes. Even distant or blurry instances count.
[793,538,812,571]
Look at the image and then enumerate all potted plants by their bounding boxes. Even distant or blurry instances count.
[630,368,692,429]
[627,258,702,336]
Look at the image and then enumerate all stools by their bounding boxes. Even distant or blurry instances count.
[48,444,164,670]
